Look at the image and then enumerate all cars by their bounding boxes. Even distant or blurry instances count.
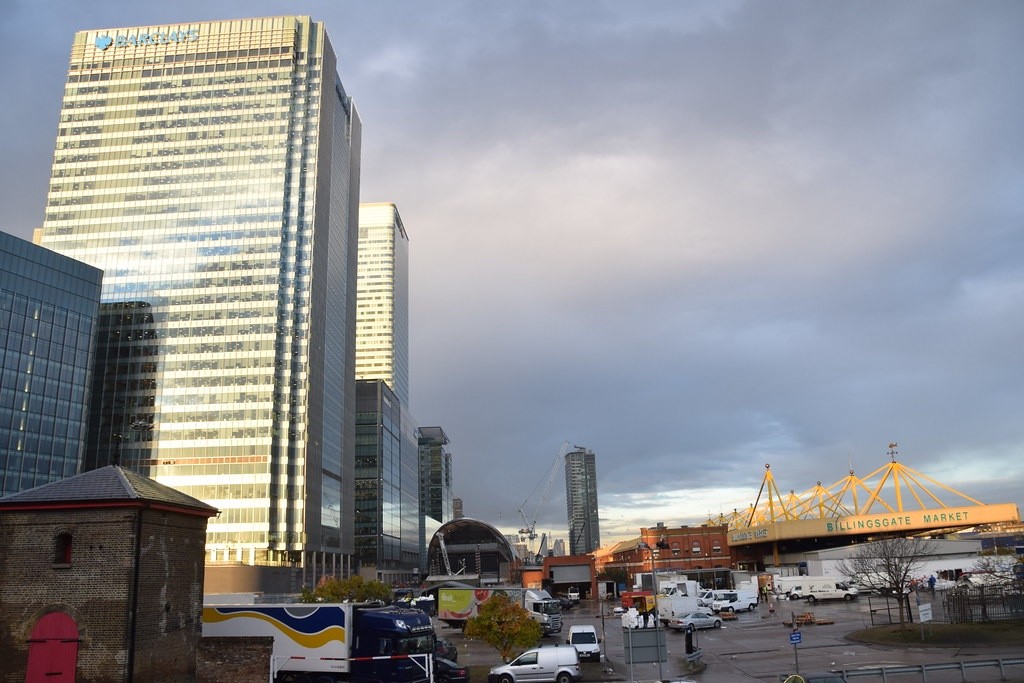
[551,597,574,609]
[668,612,722,632]
[435,657,470,683]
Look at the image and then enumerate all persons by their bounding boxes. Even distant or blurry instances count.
[912,574,936,593]
[761,585,768,600]
[643,605,659,622]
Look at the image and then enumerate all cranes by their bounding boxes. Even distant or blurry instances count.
[517,440,569,564]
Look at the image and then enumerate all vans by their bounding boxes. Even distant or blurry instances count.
[487,645,583,683]
[847,572,891,593]
[802,577,859,603]
[566,625,601,662]
[568,588,580,603]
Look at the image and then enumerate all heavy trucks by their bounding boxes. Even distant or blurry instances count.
[202,602,438,683]
[437,587,563,638]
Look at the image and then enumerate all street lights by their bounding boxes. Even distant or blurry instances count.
[638,541,670,683]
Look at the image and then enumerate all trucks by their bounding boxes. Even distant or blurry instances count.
[621,573,696,615]
[657,598,713,626]
[698,591,721,608]
[712,590,757,613]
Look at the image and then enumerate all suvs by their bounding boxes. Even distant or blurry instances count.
[436,640,458,661]
[789,585,802,599]
